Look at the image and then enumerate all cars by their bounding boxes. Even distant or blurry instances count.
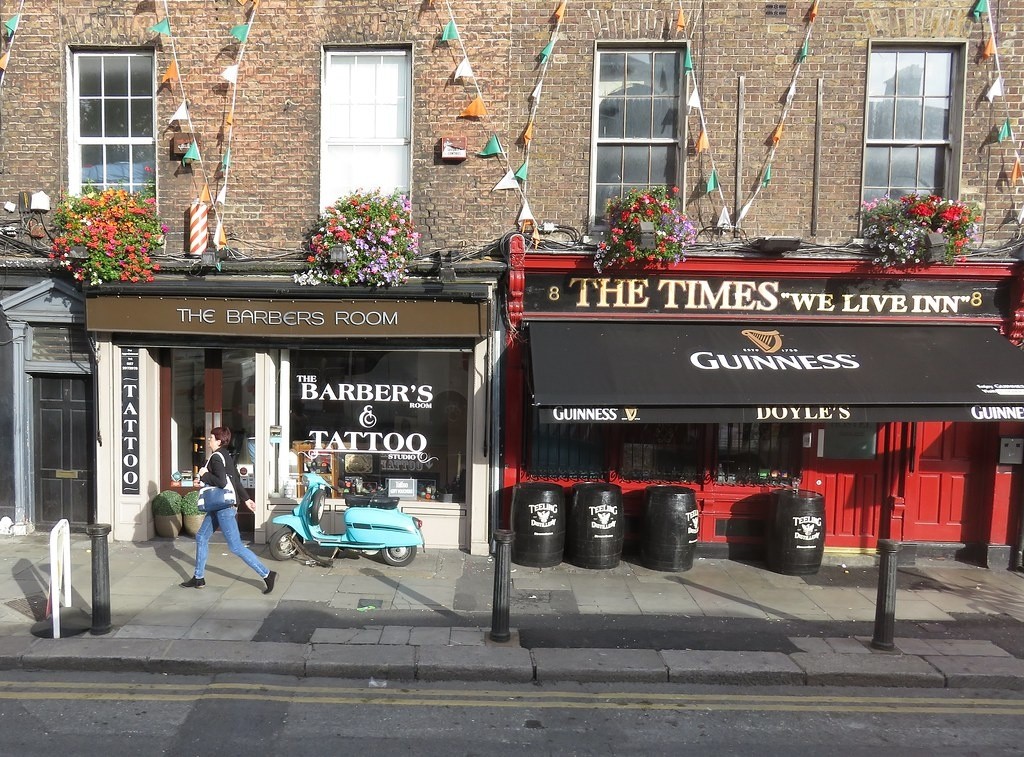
[234,435,274,488]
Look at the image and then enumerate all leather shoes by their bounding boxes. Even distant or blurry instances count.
[180,576,206,588]
[263,571,279,594]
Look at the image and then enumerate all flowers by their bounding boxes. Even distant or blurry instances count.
[593,186,697,275]
[48,164,172,287]
[291,185,423,288]
[862,193,982,271]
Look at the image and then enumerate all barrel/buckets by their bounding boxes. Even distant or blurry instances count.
[767,488,826,575]
[637,484,699,572]
[565,480,624,571]
[511,479,565,567]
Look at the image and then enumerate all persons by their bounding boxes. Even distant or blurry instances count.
[181,425,277,593]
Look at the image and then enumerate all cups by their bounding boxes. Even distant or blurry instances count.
[792,481,799,493]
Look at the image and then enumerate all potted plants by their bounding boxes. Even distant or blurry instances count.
[180,490,208,538]
[152,489,184,538]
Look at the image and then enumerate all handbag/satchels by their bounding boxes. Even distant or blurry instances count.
[197,473,236,512]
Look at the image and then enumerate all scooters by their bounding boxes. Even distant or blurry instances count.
[266,472,427,568]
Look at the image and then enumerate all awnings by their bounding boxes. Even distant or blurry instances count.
[520,317,1024,422]
